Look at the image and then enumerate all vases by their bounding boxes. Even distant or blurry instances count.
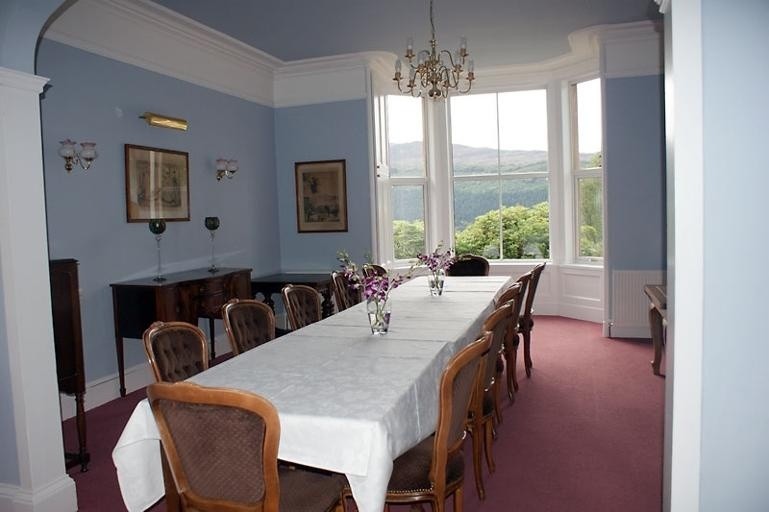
[368,296,393,336]
[428,269,447,297]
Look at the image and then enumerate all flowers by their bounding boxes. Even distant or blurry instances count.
[406,240,457,287]
[335,248,432,329]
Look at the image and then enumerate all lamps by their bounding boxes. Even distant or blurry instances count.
[393,0,476,103]
[55,140,99,171]
[216,158,241,182]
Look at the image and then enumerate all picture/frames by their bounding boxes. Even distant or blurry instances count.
[294,159,351,235]
[125,143,192,224]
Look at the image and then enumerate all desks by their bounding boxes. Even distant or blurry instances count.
[252,273,336,320]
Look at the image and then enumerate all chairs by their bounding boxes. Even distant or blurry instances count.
[146,382,343,512]
[283,283,322,333]
[510,272,534,403]
[472,300,511,501]
[509,263,546,376]
[444,253,490,277]
[143,320,213,383]
[223,300,275,357]
[495,283,521,422]
[334,331,494,512]
